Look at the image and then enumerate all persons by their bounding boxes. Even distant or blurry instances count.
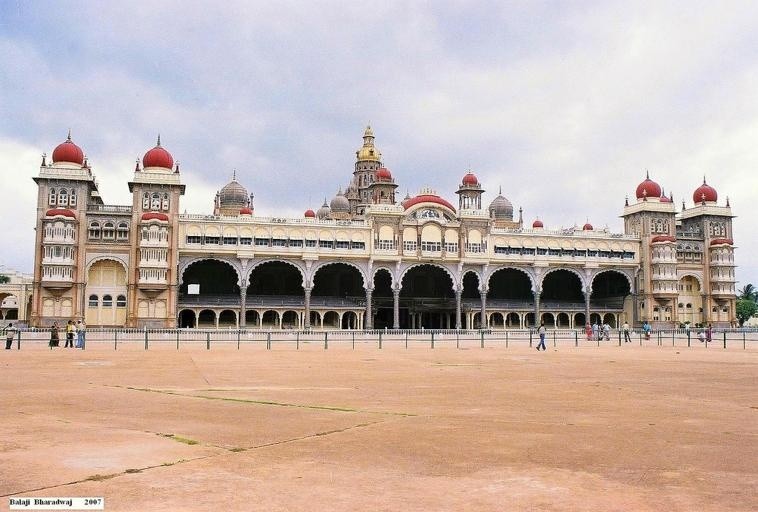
[536,324,546,351]
[49,320,60,346]
[585,322,612,341]
[2,322,17,349]
[697,331,705,342]
[70,321,78,347]
[76,320,84,348]
[621,321,631,342]
[63,319,74,348]
[706,323,712,342]
[643,320,651,340]
